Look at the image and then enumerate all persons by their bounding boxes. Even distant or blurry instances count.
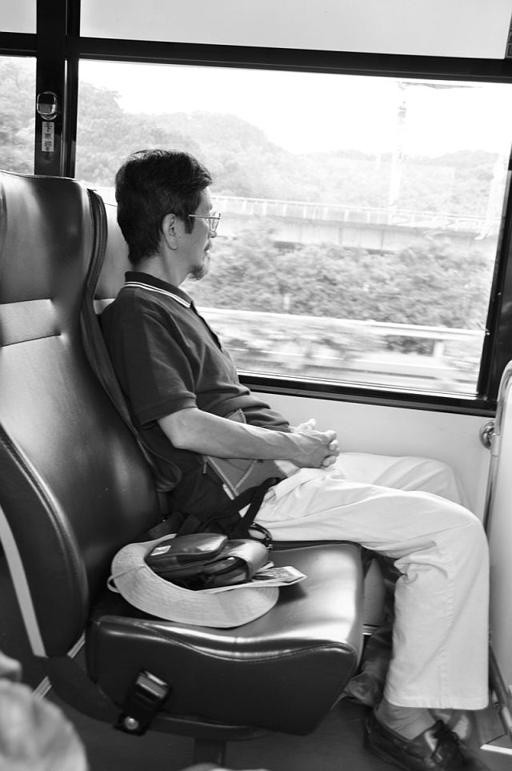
[98,149,491,771]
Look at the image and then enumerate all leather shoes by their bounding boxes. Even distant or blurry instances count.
[364,712,488,771]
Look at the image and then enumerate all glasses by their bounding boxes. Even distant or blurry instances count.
[189,213,221,231]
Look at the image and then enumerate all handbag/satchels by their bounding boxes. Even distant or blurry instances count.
[202,409,287,498]
[147,533,267,589]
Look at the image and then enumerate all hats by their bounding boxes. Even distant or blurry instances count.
[112,535,278,630]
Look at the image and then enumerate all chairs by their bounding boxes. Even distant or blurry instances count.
[0,169,396,765]
